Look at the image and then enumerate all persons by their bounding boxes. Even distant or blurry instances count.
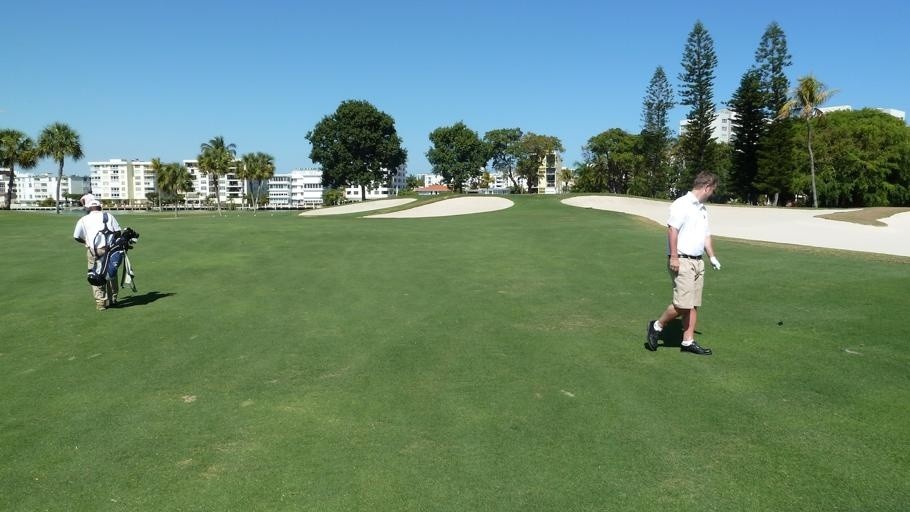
[73,200,123,310]
[80,190,95,215]
[646,171,722,355]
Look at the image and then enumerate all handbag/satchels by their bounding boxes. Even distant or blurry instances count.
[86,209,140,289]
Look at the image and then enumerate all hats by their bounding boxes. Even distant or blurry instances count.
[79,193,102,209]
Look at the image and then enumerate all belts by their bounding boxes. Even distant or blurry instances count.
[667,253,704,261]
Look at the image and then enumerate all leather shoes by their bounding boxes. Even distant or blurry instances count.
[680,340,712,355]
[647,319,664,352]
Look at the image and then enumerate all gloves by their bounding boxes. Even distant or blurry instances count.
[708,256,723,271]
